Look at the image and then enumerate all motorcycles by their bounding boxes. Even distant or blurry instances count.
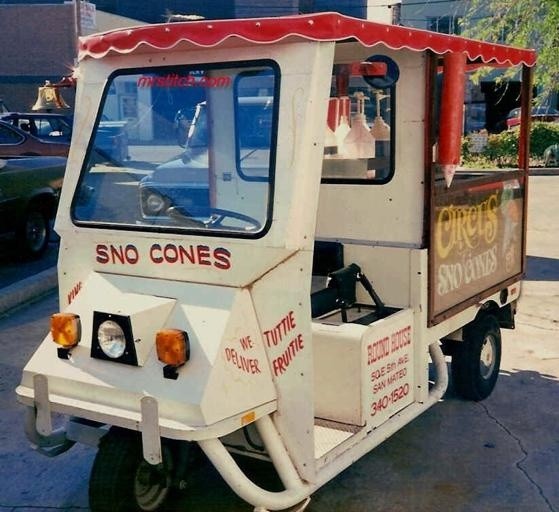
[14,11,536,511]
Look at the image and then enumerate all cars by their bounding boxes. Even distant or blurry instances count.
[504,104,558,129]
[134,94,275,225]
[2,106,128,172]
[1,154,93,255]
